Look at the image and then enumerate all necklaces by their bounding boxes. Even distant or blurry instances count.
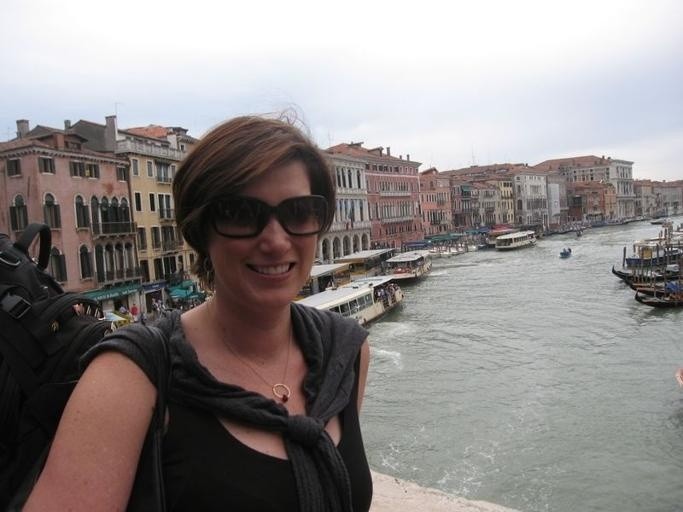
[219,325,292,402]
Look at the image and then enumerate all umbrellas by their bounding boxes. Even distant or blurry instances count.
[168,287,193,303]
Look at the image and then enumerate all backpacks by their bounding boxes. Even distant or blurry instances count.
[0,221,173,512]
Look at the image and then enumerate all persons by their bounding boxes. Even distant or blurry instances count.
[116,298,198,327]
[318,256,424,317]
[20,113,376,512]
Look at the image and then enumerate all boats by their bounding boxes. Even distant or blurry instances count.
[549,210,675,233]
[610,222,682,308]
[558,248,572,257]
[293,221,536,328]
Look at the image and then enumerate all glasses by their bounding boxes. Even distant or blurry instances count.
[208,191,330,239]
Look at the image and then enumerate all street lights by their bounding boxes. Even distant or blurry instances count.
[134,283,144,326]
